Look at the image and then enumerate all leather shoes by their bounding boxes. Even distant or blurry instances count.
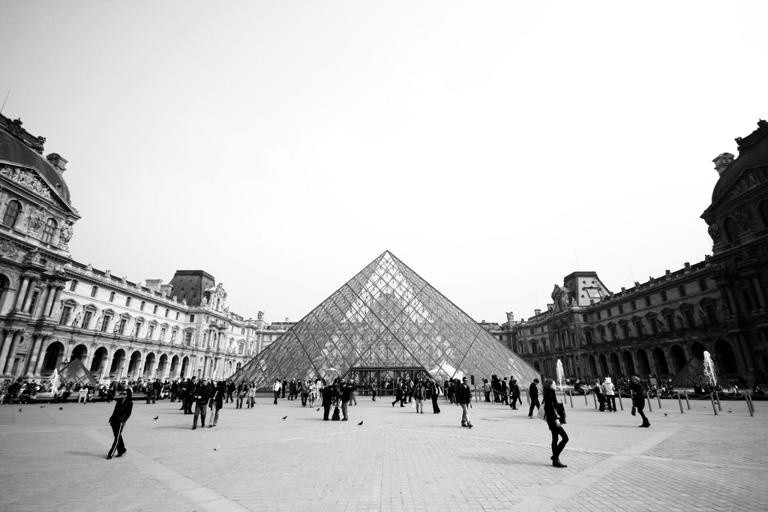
[106,448,127,460]
[551,455,568,467]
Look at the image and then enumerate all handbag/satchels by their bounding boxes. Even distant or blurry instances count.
[631,406,636,416]
[331,406,341,422]
[551,402,566,426]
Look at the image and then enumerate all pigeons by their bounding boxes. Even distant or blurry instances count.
[16,404,64,413]
[316,407,320,411]
[356,420,363,425]
[153,415,158,420]
[281,415,287,421]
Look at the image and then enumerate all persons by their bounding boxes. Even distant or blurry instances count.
[107,389,133,459]
[0,375,722,430]
[543,379,570,468]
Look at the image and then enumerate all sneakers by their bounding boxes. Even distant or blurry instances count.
[192,423,216,430]
